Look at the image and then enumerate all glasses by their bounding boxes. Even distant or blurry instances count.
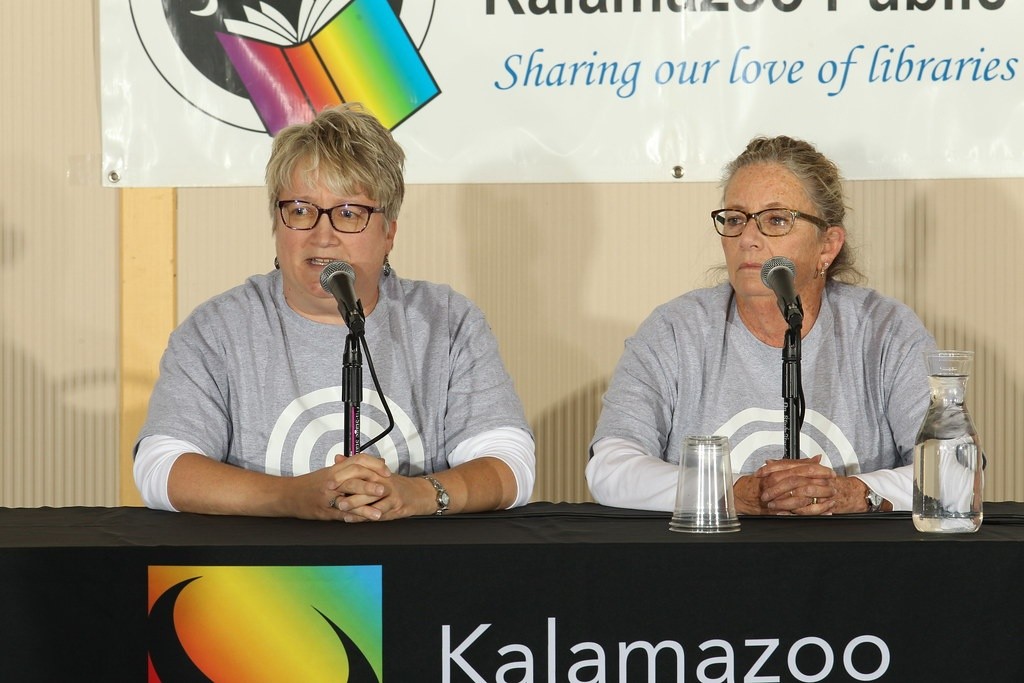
[276,199,385,233]
[711,208,831,237]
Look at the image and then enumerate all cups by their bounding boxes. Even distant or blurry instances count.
[669,435,742,534]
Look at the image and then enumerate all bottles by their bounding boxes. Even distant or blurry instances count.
[912,350,984,533]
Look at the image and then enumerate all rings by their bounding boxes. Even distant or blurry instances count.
[328,494,347,507]
[812,497,817,504]
[790,489,794,497]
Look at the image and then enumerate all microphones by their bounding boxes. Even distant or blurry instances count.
[761,256,803,331]
[320,261,365,336]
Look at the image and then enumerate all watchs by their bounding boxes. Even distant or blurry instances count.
[421,475,450,516]
[865,488,883,513]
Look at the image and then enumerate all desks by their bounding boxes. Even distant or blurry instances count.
[1,504,1024,683]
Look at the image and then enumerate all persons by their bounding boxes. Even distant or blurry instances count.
[133,102,538,523]
[585,135,986,516]
